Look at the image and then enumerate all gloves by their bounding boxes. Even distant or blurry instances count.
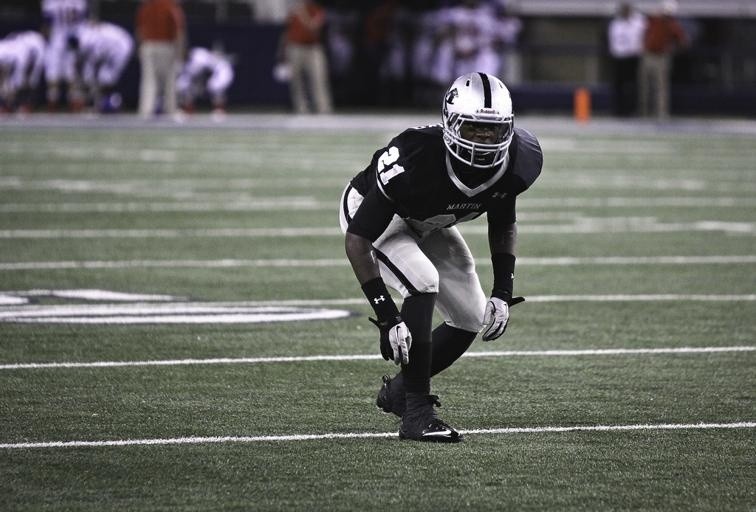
[483,297,526,340]
[369,313,414,364]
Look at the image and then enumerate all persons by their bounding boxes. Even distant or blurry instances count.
[419,0,521,89]
[284,0,332,115]
[0,0,234,118]
[338,72,542,442]
[608,0,688,123]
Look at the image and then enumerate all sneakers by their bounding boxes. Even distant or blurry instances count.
[376,375,460,441]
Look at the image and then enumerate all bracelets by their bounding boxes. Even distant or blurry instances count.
[358,276,399,321]
[488,252,516,295]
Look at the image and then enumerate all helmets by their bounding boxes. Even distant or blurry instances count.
[443,72,515,171]
